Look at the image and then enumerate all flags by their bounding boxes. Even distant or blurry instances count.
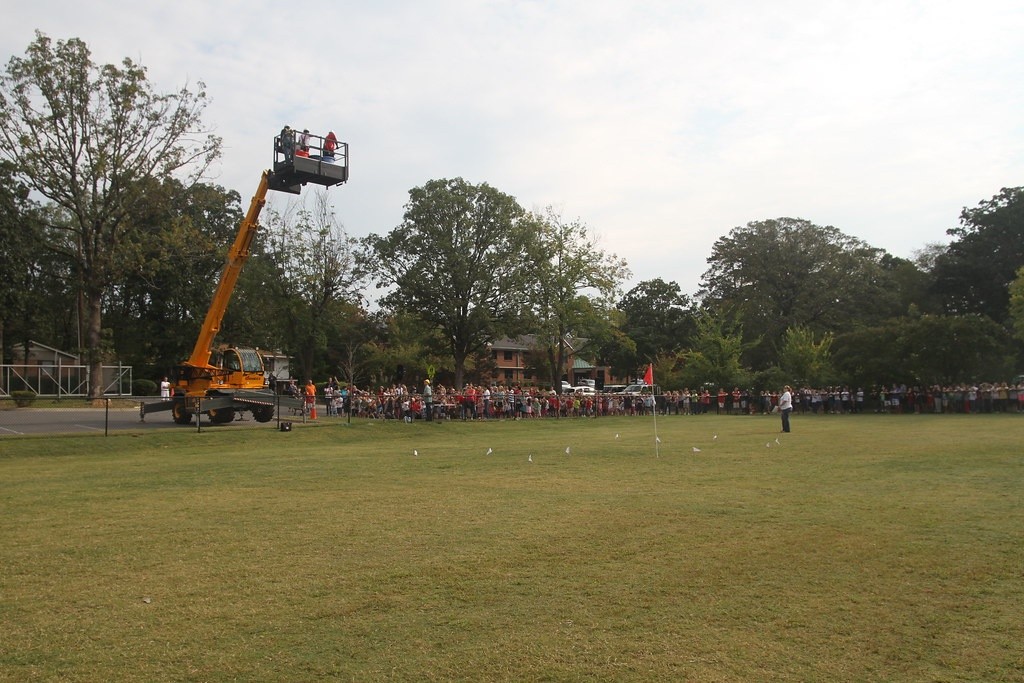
[643,366,653,385]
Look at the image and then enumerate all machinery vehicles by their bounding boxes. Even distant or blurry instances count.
[170,124,349,427]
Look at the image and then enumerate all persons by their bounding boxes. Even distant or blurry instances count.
[779,385,792,432]
[281,125,339,160]
[288,376,298,410]
[324,378,1024,421]
[305,379,317,411]
[160,377,170,402]
[268,373,278,394]
